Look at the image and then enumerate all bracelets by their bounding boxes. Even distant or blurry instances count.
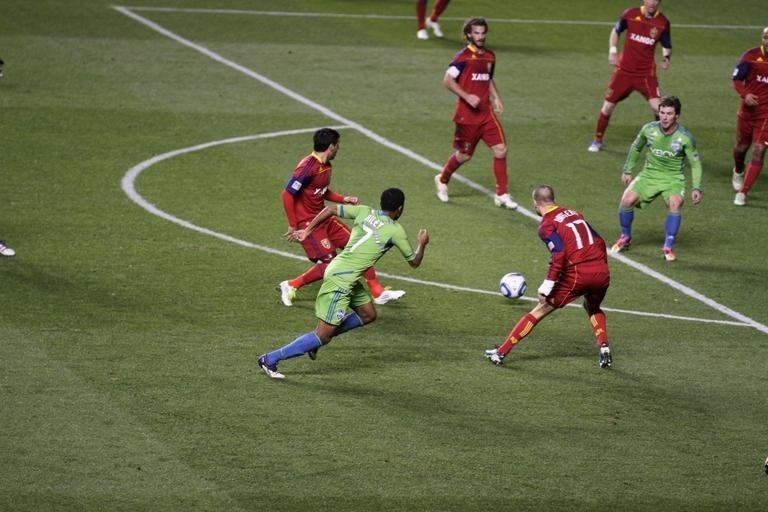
[608,45,616,54]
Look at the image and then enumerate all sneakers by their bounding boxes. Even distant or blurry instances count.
[0,242,15,257]
[732,166,745,192]
[434,173,449,203]
[371,285,406,305]
[257,280,319,379]
[599,345,613,367]
[733,191,748,206]
[417,29,428,41]
[484,344,505,365]
[611,234,632,253]
[425,18,444,39]
[494,193,518,209]
[662,244,675,262]
[588,141,603,153]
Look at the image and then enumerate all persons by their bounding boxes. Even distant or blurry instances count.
[583,0,672,154]
[609,97,706,264]
[256,187,429,380]
[482,184,613,367]
[730,27,768,207]
[434,18,518,210]
[413,0,448,40]
[0,236,17,259]
[276,127,407,308]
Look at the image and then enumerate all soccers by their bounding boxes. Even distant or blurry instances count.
[499,273,527,299]
